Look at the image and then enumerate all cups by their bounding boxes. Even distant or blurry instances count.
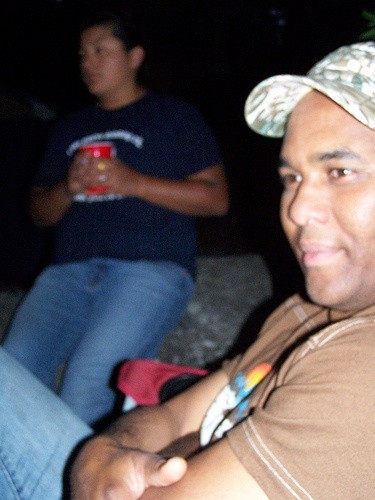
[77,141,116,196]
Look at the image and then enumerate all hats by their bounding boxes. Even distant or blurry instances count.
[243,40,375,138]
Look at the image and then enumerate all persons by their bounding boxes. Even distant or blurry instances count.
[1,12,230,426]
[1,42,375,500]
